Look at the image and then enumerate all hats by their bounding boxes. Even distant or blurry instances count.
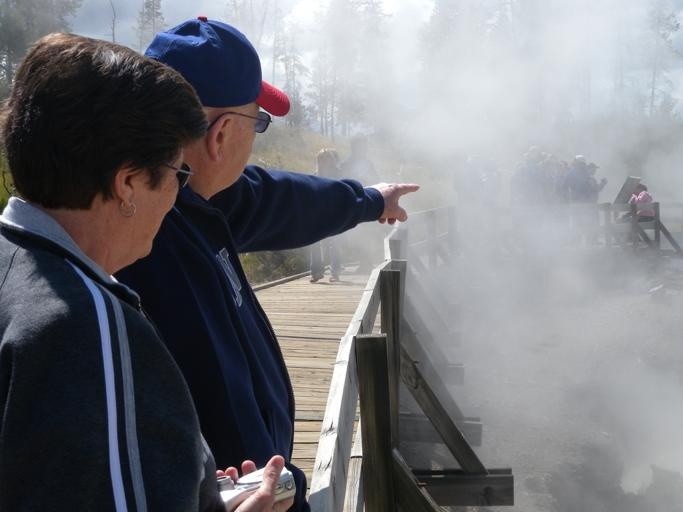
[144,16,289,116]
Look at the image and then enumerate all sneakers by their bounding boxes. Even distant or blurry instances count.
[311,275,338,282]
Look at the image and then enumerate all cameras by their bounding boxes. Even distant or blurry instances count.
[216,465,297,512]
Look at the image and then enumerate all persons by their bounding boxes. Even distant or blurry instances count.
[304,128,657,282]
[0,31,298,509]
[103,13,421,509]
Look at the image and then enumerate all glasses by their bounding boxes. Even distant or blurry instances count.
[163,163,194,189]
[206,111,273,133]
